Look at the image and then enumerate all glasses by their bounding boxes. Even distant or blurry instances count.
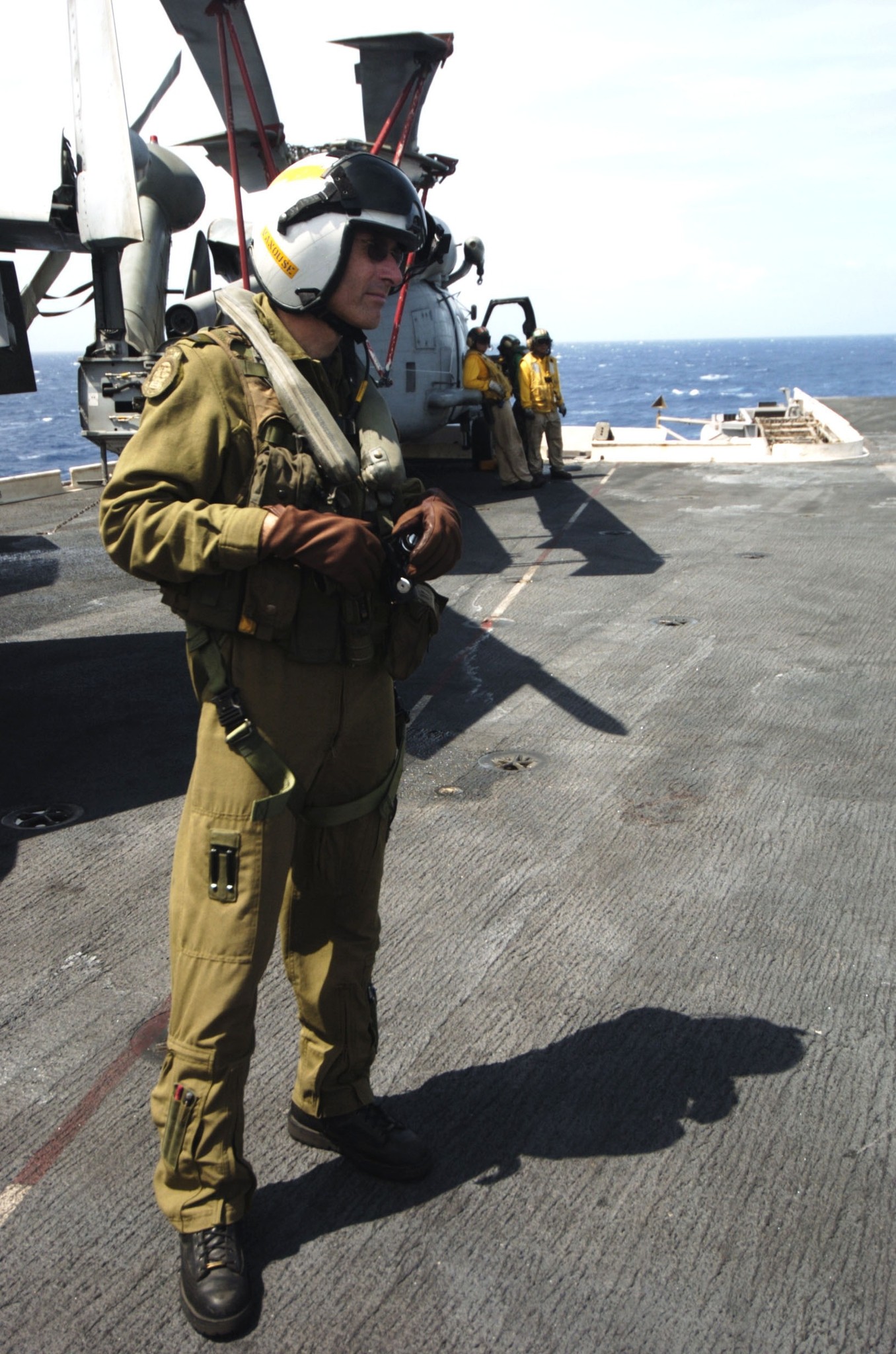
[274,153,428,235]
[359,238,405,264]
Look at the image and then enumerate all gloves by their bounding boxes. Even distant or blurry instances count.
[489,379,502,393]
[261,502,386,600]
[391,487,463,581]
[525,408,536,420]
[558,403,567,416]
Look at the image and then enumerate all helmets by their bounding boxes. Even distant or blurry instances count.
[467,326,489,347]
[498,334,520,353]
[245,149,427,312]
[533,329,549,343]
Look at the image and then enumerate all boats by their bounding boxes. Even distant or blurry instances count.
[700,400,839,447]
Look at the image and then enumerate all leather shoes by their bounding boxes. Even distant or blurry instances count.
[287,1097,433,1182]
[178,1225,254,1335]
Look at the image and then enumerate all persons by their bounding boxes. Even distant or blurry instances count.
[518,328,572,480]
[462,326,541,490]
[98,152,466,1341]
[496,335,530,459]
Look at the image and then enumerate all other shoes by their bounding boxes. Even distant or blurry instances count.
[533,467,572,485]
[497,477,539,489]
